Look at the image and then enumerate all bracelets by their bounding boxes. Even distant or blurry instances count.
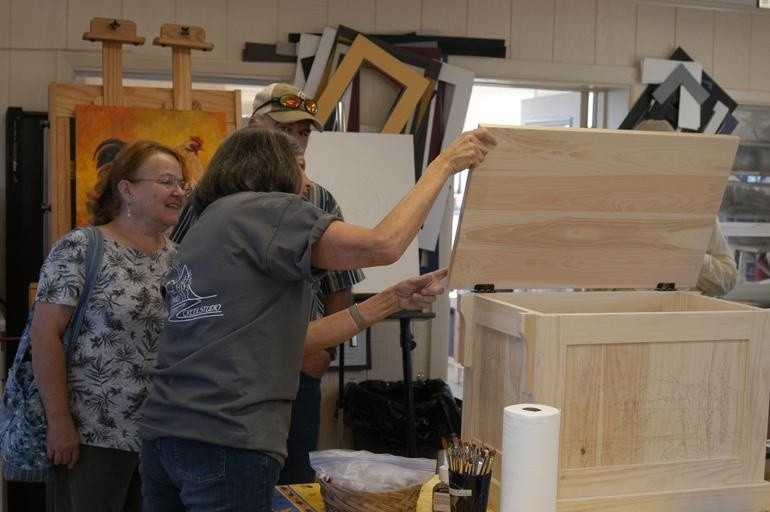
[349,306,369,331]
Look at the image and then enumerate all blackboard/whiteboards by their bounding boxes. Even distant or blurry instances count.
[303,130,420,294]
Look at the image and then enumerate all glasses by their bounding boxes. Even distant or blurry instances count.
[131,176,193,196]
[253,93,319,117]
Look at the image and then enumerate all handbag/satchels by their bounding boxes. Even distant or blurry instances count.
[0,362,57,482]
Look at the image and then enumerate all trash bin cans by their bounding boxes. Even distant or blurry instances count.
[347,379,452,457]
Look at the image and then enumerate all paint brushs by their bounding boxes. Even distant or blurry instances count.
[441,436,495,475]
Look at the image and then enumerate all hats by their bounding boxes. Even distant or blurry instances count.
[250,82,324,131]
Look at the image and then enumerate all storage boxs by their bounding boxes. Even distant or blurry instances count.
[444,122,770,512]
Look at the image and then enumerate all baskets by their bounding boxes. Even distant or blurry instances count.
[317,472,424,511]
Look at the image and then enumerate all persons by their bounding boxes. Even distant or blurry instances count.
[167,83,365,451]
[30,141,188,512]
[135,124,497,512]
[633,120,737,297]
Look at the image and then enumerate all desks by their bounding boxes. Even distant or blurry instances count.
[270,472,497,512]
